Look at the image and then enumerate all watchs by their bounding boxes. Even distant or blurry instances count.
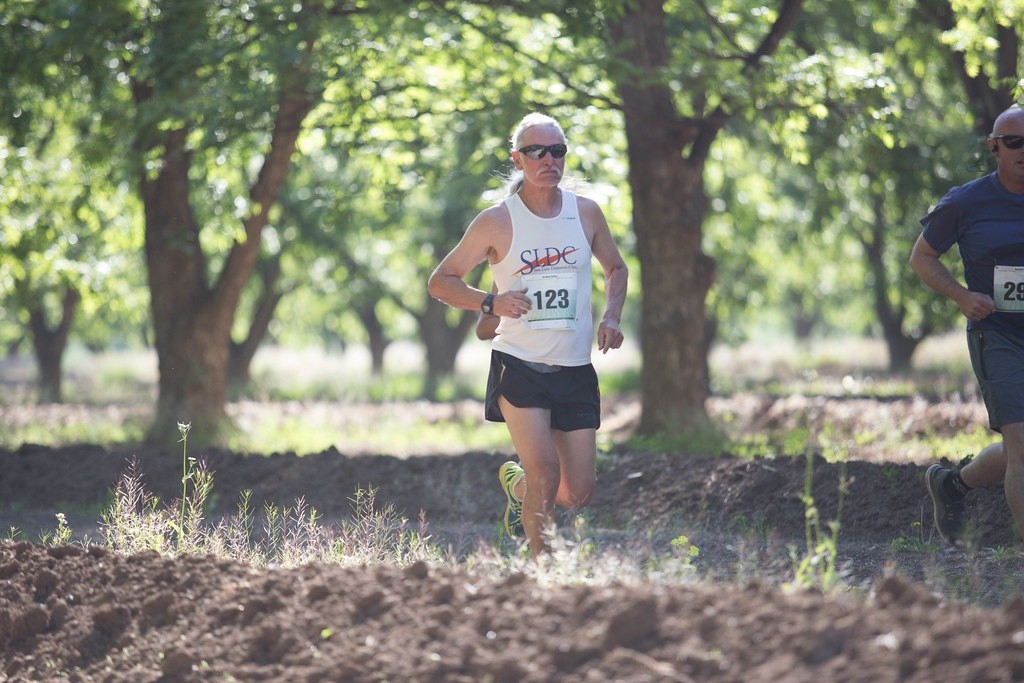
[480,294,495,315]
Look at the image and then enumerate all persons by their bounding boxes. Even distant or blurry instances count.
[908,107,1024,545]
[427,111,628,563]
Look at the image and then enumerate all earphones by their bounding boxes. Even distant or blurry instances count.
[992,145,998,152]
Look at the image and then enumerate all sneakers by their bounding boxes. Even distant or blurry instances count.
[925,464,968,546]
[499,460,526,540]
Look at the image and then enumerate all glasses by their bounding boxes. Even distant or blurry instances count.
[518,144,567,159]
[992,134,1024,149]
